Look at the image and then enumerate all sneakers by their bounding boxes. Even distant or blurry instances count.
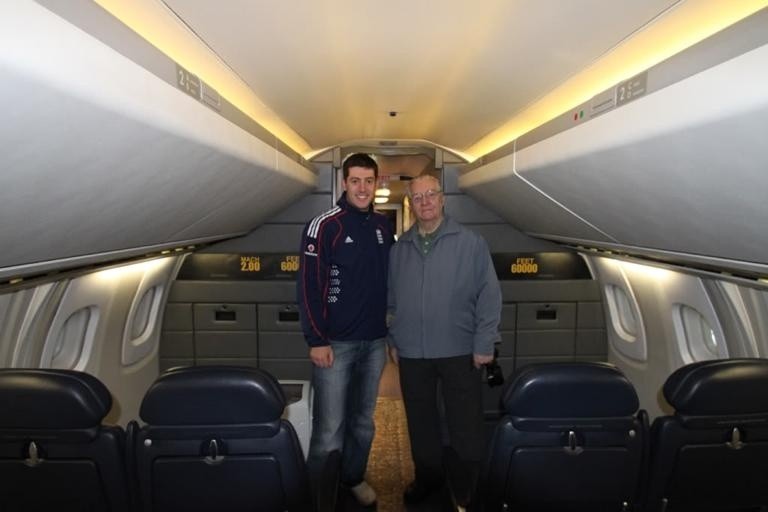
[346,478,377,507]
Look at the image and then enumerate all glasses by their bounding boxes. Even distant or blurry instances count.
[409,188,445,203]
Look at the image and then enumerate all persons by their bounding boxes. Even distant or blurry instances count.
[382,173,504,512]
[295,151,399,510]
